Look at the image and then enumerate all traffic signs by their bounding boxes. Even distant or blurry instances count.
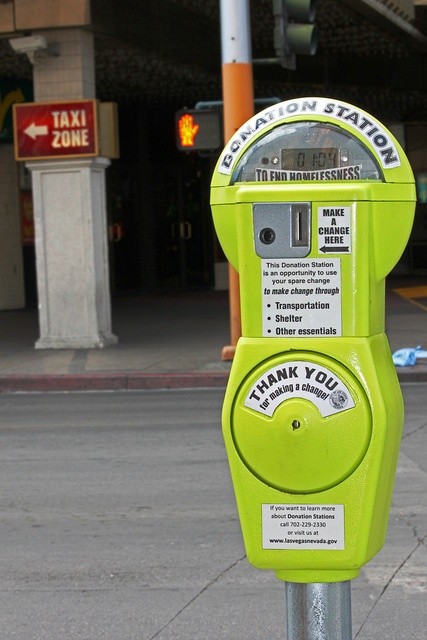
[13,107,93,160]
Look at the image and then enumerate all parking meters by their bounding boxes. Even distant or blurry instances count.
[208,95,415,640]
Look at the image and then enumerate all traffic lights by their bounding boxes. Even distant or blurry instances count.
[174,110,222,150]
